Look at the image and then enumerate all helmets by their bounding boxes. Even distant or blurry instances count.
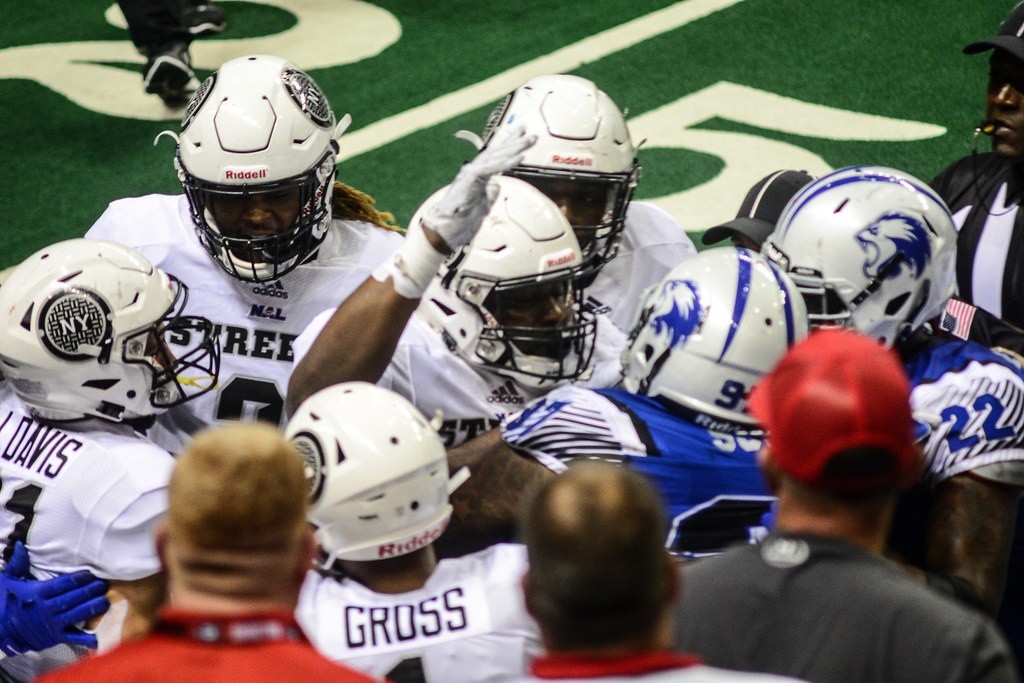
[398,175,612,388]
[761,165,960,350]
[284,382,453,560]
[172,54,340,284]
[0,238,220,427]
[481,74,642,275]
[620,246,808,425]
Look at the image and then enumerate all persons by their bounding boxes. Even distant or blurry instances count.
[0,0,1024,683]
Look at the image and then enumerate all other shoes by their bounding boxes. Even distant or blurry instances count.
[140,43,201,109]
[185,4,227,34]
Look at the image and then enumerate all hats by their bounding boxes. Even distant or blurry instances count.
[963,1,1024,60]
[701,169,814,248]
[747,330,915,489]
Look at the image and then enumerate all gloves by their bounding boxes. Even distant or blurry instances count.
[419,124,538,254]
[0,542,110,656]
